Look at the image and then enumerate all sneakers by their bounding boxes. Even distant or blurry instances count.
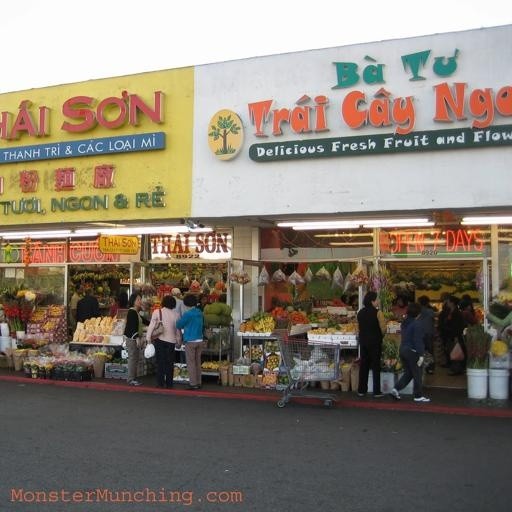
[412,396,432,402]
[128,379,202,390]
[358,393,384,398]
[390,388,401,401]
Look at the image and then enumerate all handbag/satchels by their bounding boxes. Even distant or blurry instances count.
[150,309,164,337]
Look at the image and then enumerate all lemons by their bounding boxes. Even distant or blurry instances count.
[263,373,277,384]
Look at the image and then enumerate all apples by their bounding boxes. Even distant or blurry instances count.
[272,307,289,320]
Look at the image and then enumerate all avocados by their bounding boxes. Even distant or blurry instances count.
[279,376,288,384]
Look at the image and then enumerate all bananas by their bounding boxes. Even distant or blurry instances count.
[255,315,275,332]
[73,271,124,280]
[154,267,183,281]
[201,361,220,370]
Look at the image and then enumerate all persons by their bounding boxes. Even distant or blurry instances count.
[392,302,430,402]
[197,293,212,312]
[357,292,387,399]
[70,284,128,336]
[388,292,480,373]
[146,295,182,388]
[341,295,359,310]
[123,293,150,386]
[295,282,311,302]
[171,287,185,364]
[176,295,205,389]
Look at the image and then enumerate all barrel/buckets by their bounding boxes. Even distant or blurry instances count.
[0,337,12,353]
[489,369,510,400]
[466,368,488,399]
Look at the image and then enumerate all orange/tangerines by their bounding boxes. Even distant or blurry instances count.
[191,282,199,290]
[152,303,160,312]
[291,311,308,324]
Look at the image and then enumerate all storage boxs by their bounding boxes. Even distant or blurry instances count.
[308,306,358,347]
[220,364,263,388]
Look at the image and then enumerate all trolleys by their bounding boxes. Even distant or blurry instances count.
[268,331,343,409]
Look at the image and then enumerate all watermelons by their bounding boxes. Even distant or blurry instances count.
[203,302,232,326]
